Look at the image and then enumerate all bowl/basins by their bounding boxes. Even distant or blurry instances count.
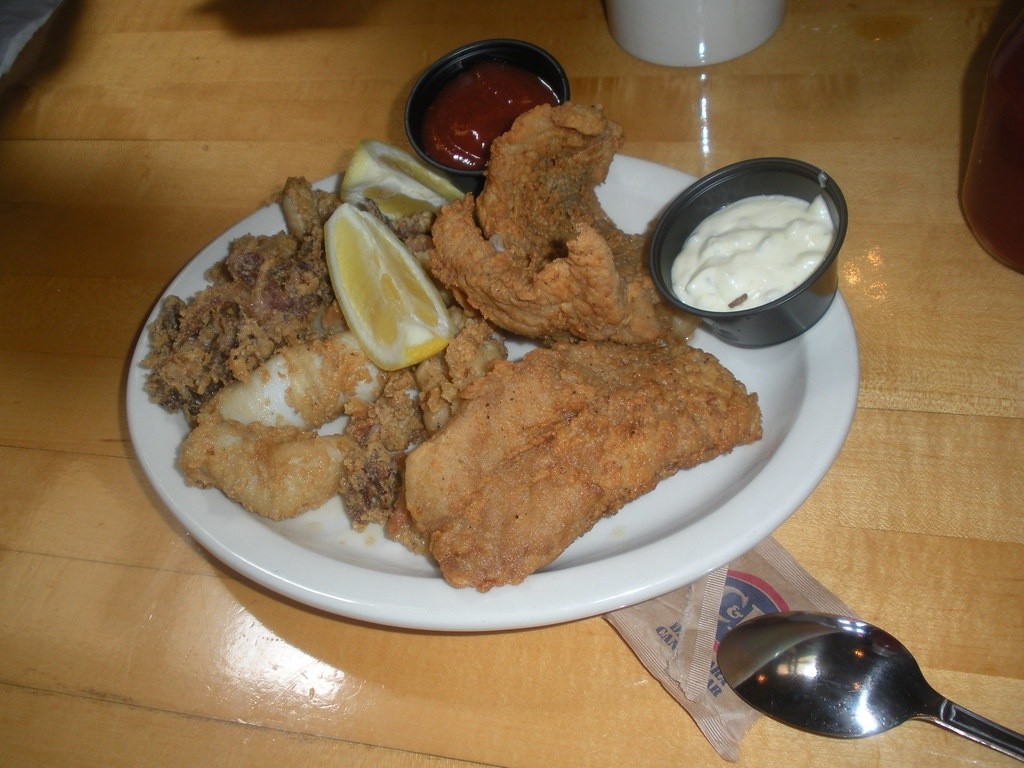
[404,37,571,176]
[649,157,849,348]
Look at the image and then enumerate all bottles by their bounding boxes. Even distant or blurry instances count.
[961,4,1024,275]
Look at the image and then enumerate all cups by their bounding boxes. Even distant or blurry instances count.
[605,0,785,66]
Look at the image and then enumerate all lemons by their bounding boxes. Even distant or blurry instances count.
[342,139,465,219]
[325,204,453,372]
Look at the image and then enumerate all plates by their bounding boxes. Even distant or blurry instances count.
[126,156,859,632]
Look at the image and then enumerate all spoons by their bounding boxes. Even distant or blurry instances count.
[718,611,1023,768]
